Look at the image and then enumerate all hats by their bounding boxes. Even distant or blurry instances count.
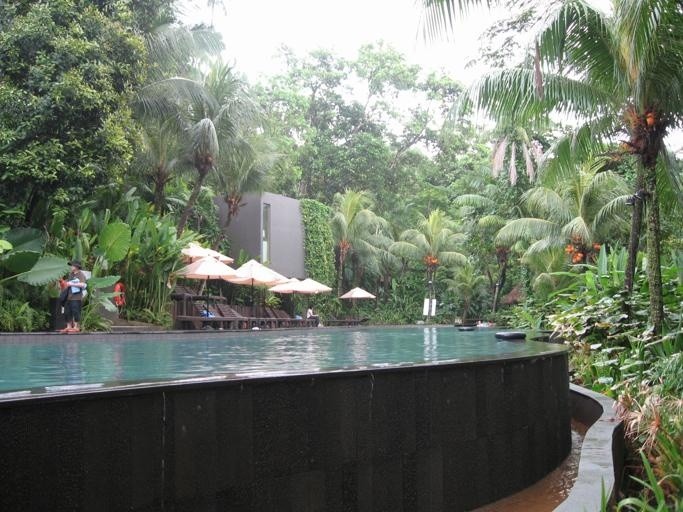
[68,259,83,269]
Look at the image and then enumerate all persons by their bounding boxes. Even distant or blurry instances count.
[487,319,495,327]
[475,319,484,327]
[57,339,86,387]
[306,305,323,328]
[57,258,87,332]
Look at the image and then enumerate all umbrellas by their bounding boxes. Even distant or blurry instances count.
[176,242,219,264]
[172,255,245,317]
[290,277,332,316]
[267,277,318,317]
[202,247,234,265]
[220,259,292,317]
[339,287,376,319]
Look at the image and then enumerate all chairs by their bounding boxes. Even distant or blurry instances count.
[327,318,370,326]
[194,301,317,327]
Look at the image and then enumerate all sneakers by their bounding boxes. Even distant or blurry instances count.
[61,328,69,332]
[68,328,80,332]
